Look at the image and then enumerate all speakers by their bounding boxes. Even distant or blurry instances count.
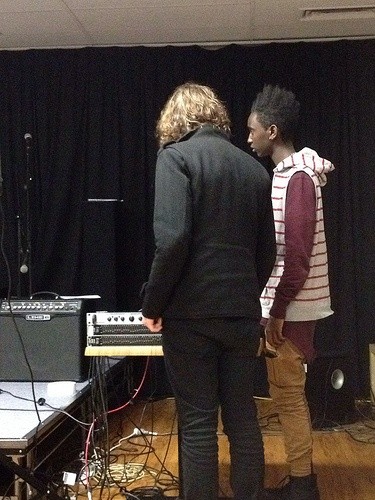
[0,291,97,383]
[304,353,356,429]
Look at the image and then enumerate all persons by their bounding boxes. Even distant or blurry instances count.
[247,83,335,500]
[139,82,278,500]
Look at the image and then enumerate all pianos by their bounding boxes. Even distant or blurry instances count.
[84,310,164,357]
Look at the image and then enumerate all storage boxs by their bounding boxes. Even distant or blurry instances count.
[0,355,133,500]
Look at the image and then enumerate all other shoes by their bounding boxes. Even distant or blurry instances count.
[263,474,321,500]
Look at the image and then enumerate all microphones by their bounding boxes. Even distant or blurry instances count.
[24,133,33,156]
[19,248,29,273]
